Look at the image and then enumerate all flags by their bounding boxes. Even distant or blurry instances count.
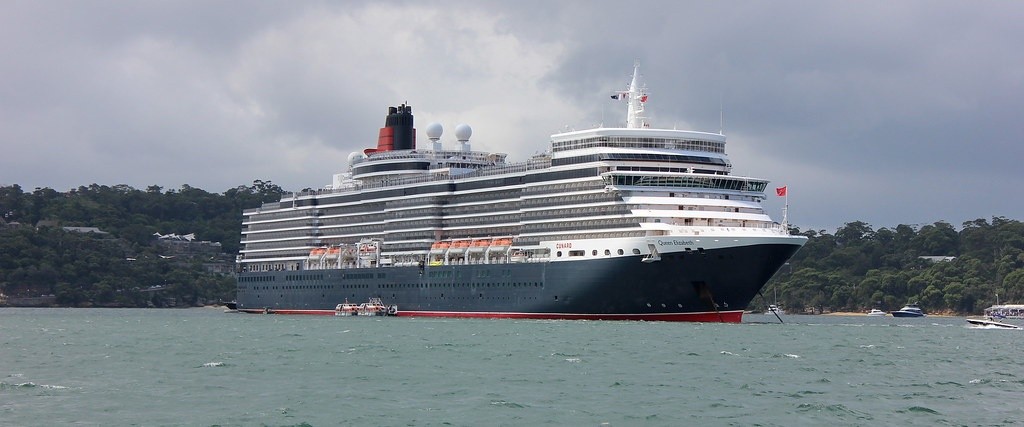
[610,94,625,101]
[641,96,648,102]
[776,186,786,197]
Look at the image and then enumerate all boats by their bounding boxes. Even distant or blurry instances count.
[867,309,887,318]
[309,245,376,262]
[430,240,513,258]
[966,318,1017,329]
[982,314,1006,320]
[764,301,785,315]
[357,297,388,317]
[890,301,926,317]
[334,298,359,317]
[222,301,237,310]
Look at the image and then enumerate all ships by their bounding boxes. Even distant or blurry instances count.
[218,60,811,323]
